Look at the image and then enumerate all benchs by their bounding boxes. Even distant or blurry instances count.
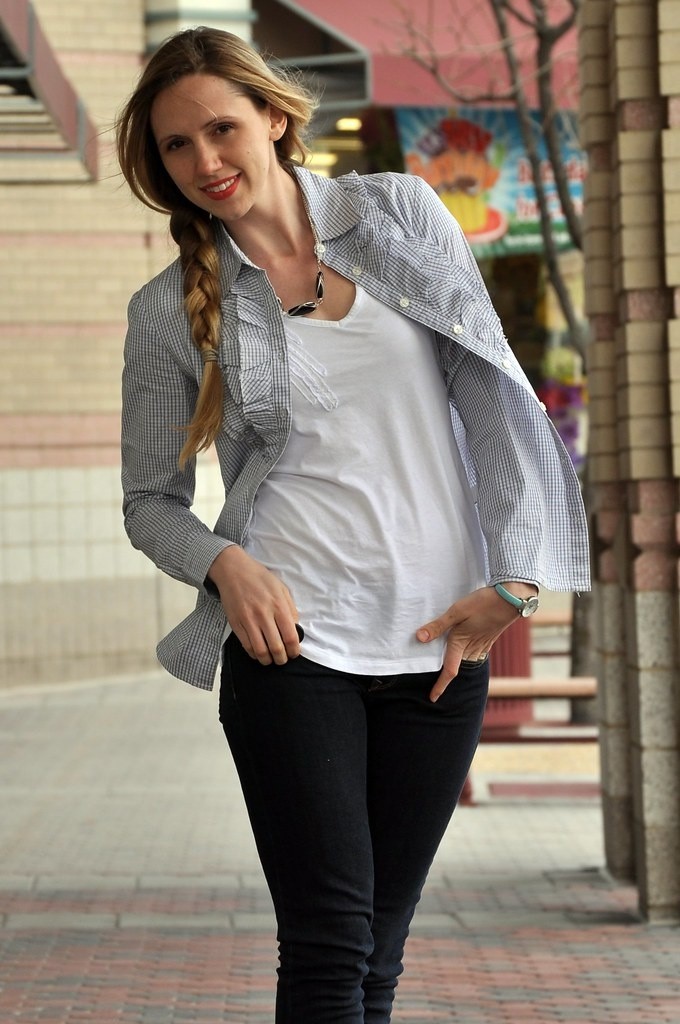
[480,677,600,746]
[527,612,574,658]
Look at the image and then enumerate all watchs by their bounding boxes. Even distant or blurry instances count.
[494,583,540,618]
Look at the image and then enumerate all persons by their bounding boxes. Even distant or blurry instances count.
[116,26,595,1024]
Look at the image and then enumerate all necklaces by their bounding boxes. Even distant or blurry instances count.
[277,177,327,317]
